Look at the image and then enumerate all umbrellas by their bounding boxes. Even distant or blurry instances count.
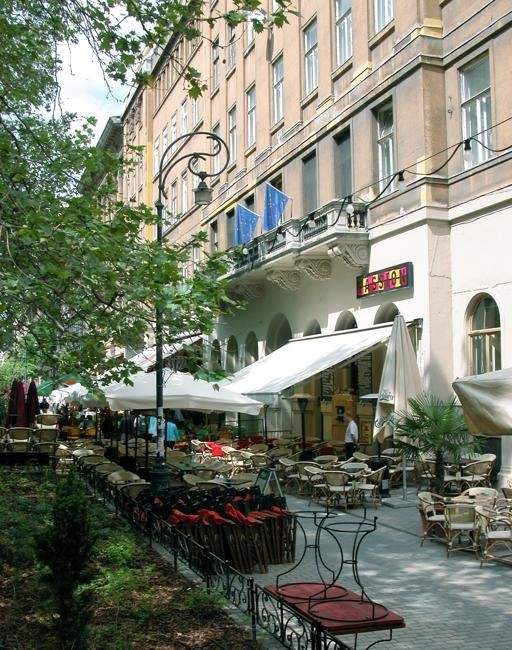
[5,367,264,465]
[375,311,437,509]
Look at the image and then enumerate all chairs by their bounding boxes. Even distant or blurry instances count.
[0,412,512,649]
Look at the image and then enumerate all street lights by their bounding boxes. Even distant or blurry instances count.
[155,129,233,469]
[22,335,38,395]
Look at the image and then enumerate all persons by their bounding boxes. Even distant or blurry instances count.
[343,410,360,459]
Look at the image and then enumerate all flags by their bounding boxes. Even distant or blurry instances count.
[233,205,260,248]
[263,185,289,231]
